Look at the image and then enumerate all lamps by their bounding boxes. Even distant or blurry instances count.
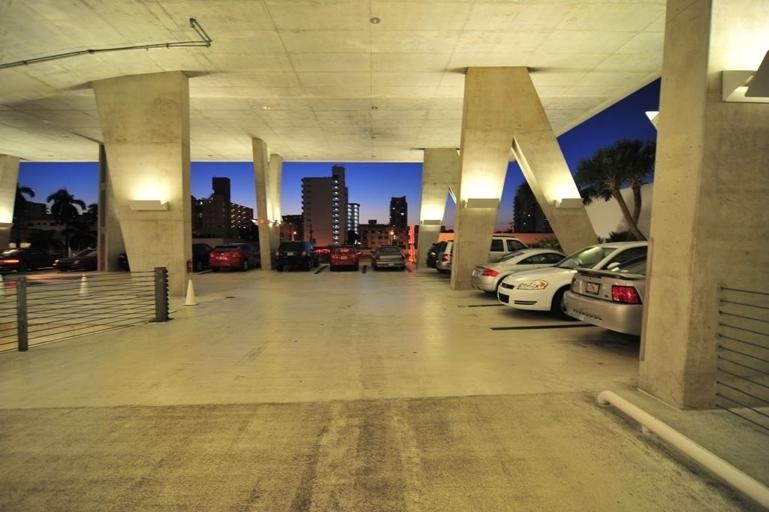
[721,68,769,104]
[644,111,659,131]
[463,198,500,208]
[745,50,769,98]
[0,222,15,230]
[422,220,442,226]
[555,198,584,209]
[127,199,169,211]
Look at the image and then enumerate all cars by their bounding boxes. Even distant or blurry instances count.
[426,237,649,336]
[193,243,258,269]
[0,248,129,272]
[275,241,405,271]
